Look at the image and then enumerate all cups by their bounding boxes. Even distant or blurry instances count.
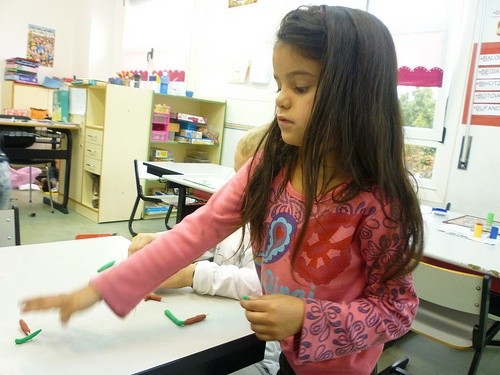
[186,91,193,97]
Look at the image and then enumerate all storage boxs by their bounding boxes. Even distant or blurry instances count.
[168,113,215,146]
[5,57,39,85]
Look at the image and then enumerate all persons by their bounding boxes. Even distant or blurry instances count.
[17,4,425,375]
[128,125,283,375]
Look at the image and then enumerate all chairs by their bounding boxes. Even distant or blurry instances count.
[128,161,197,237]
[407,259,500,375]
[0,115,54,213]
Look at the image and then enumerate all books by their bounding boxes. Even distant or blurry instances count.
[5,55,39,83]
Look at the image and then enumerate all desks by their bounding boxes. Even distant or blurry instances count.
[0,118,80,215]
[143,162,500,375]
[0,236,266,375]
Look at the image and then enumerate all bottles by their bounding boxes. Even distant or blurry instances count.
[160,76,168,94]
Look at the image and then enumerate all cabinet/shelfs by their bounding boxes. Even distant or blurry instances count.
[142,92,227,220]
[58,80,154,224]
[0,80,56,169]
